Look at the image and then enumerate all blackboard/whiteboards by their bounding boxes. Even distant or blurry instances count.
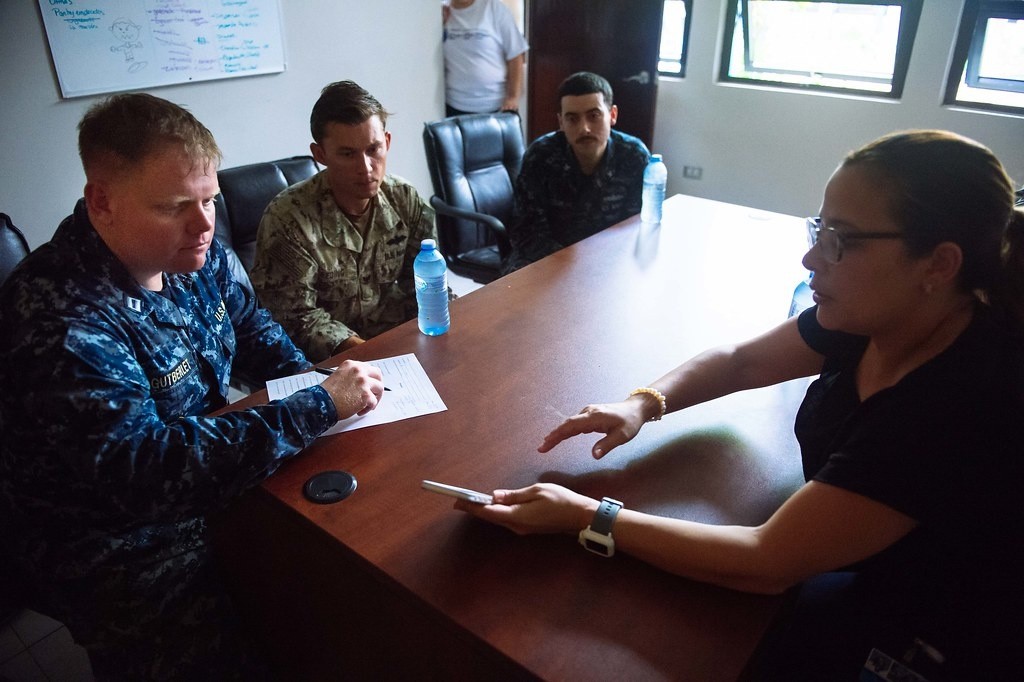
[38,0,284,99]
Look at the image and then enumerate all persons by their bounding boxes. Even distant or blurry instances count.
[453,127,1024,682]
[247,80,439,364]
[496,72,653,278]
[0,92,385,682]
[444,0,528,112]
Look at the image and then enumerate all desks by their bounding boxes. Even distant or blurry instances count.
[213,193,821,682]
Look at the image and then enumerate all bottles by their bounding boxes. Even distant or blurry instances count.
[789,271,815,318]
[640,154,667,223]
[414,239,450,337]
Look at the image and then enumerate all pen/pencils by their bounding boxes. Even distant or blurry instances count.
[316,366,392,391]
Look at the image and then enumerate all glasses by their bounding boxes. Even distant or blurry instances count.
[806,216,912,265]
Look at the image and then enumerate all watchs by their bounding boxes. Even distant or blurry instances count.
[577,496,624,558]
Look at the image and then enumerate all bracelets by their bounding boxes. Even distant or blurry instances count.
[632,387,666,423]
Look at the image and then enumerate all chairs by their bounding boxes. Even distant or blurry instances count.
[423,109,527,283]
[0,211,32,287]
[216,154,322,270]
